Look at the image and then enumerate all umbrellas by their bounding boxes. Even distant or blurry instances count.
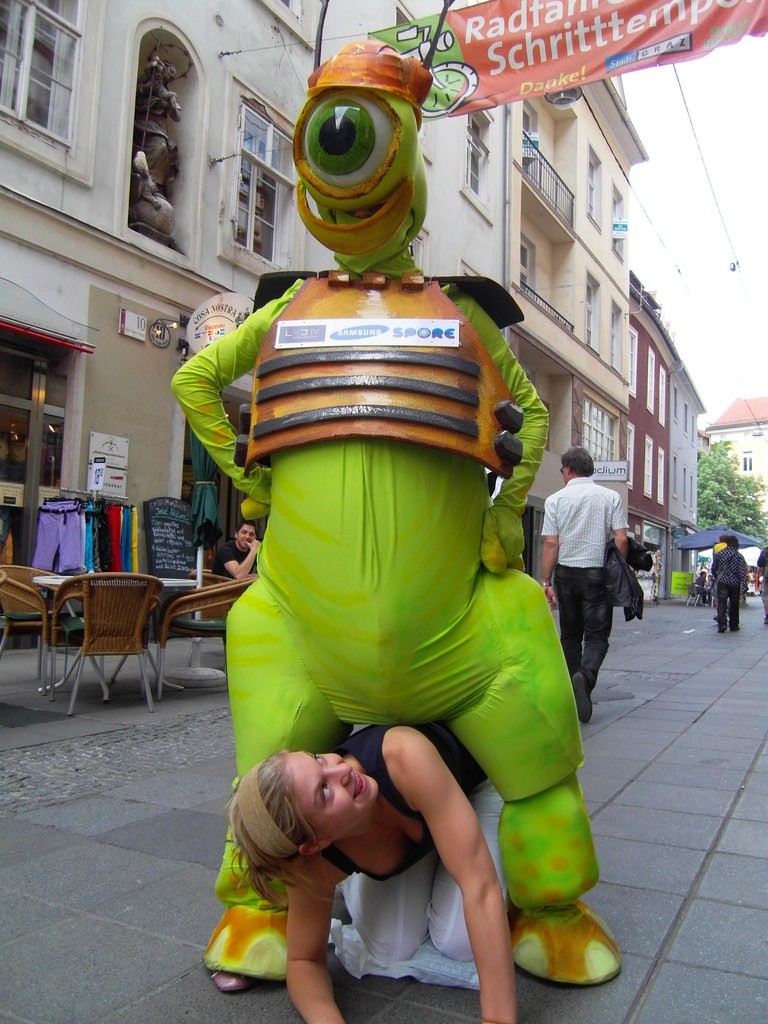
[698,546,762,566]
[188,426,219,622]
[675,522,761,550]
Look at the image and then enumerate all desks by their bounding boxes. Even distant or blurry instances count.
[32,574,198,702]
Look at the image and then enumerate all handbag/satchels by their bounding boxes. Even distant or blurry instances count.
[711,581,718,599]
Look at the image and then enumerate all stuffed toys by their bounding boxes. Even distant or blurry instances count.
[170,0,619,995]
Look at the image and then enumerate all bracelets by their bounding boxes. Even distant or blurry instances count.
[543,582,552,586]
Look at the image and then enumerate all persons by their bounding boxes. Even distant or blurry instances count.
[711,533,748,633]
[210,522,263,580]
[757,546,768,624]
[540,447,630,724]
[228,724,518,1024]
[695,571,711,607]
[136,56,182,195]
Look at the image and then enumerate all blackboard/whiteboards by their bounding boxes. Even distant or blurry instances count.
[143,496,197,578]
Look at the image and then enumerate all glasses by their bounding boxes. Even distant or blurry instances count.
[560,464,568,473]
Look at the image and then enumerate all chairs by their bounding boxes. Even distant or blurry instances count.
[0,564,258,714]
[685,584,728,608]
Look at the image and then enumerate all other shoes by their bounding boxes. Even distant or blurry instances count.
[730,627,740,631]
[571,672,593,724]
[764,619,768,624]
[718,628,726,633]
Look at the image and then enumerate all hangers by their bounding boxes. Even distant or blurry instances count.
[0,451,26,469]
[40,487,136,511]
